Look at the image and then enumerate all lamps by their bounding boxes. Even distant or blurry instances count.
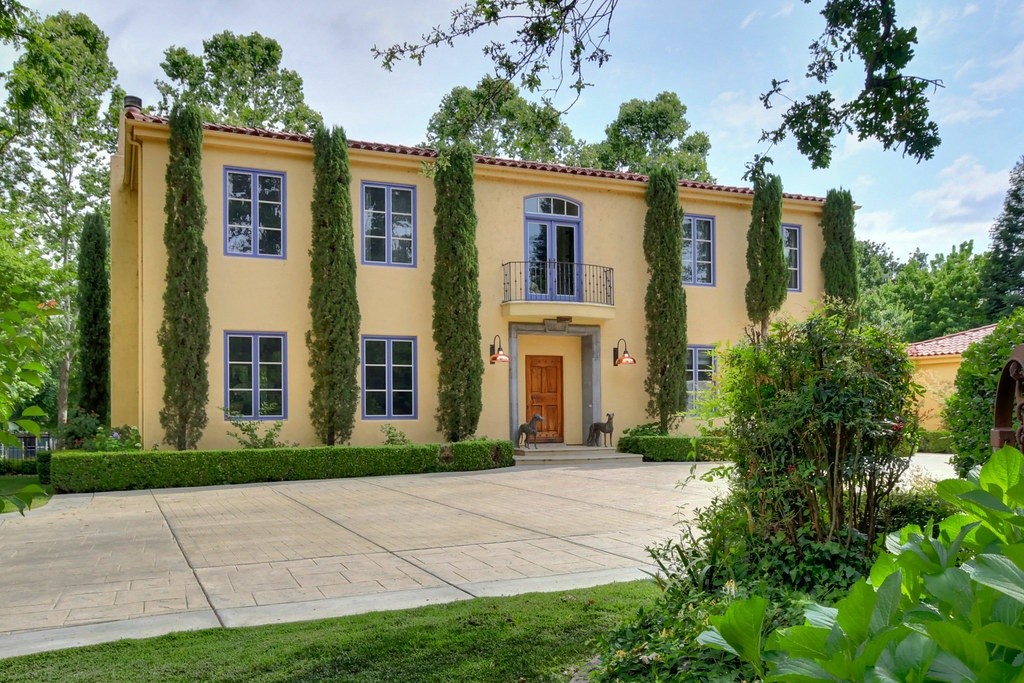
[490,335,511,364]
[613,339,636,367]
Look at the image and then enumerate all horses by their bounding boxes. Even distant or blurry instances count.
[587,413,615,448]
[517,413,543,450]
